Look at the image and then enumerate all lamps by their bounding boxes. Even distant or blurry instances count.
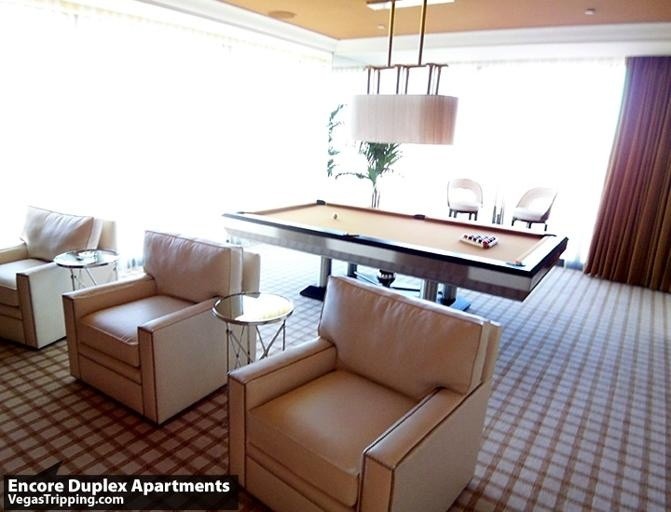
[351,0,458,143]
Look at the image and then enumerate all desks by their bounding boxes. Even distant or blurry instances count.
[54,249,120,290]
[212,292,295,372]
[221,199,568,312]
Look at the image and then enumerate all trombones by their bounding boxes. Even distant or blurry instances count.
[463,234,498,243]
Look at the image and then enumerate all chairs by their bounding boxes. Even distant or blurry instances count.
[511,188,557,231]
[63,227,263,422]
[227,275,501,512]
[448,178,483,220]
[0,205,118,349]
